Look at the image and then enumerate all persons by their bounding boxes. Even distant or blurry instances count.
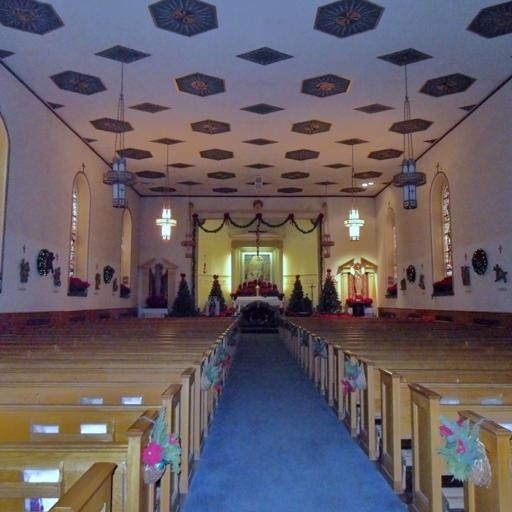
[354,263,365,295]
[149,263,168,299]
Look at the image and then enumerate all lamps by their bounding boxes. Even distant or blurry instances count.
[182,185,195,258]
[103,59,136,207]
[156,141,176,240]
[394,52,427,211]
[319,183,334,258]
[344,142,364,240]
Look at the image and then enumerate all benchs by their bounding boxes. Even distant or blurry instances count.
[278,315,512,511]
[0,315,240,511]
